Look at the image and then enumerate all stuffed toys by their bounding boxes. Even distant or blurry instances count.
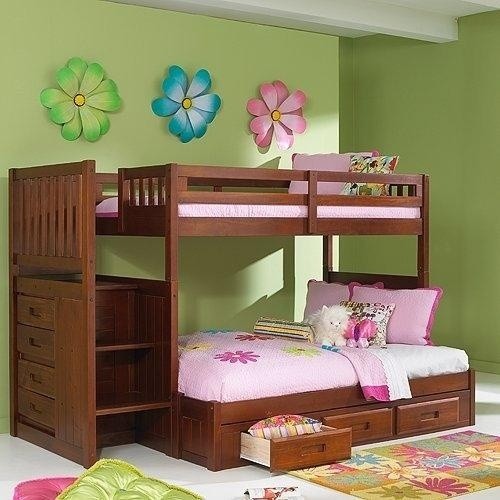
[305,304,354,348]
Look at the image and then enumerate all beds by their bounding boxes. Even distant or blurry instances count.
[4,164,476,473]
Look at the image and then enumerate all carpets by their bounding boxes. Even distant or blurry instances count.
[278,430,500,496]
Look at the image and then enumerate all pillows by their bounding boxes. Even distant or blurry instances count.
[252,276,444,352]
[286,149,400,202]
